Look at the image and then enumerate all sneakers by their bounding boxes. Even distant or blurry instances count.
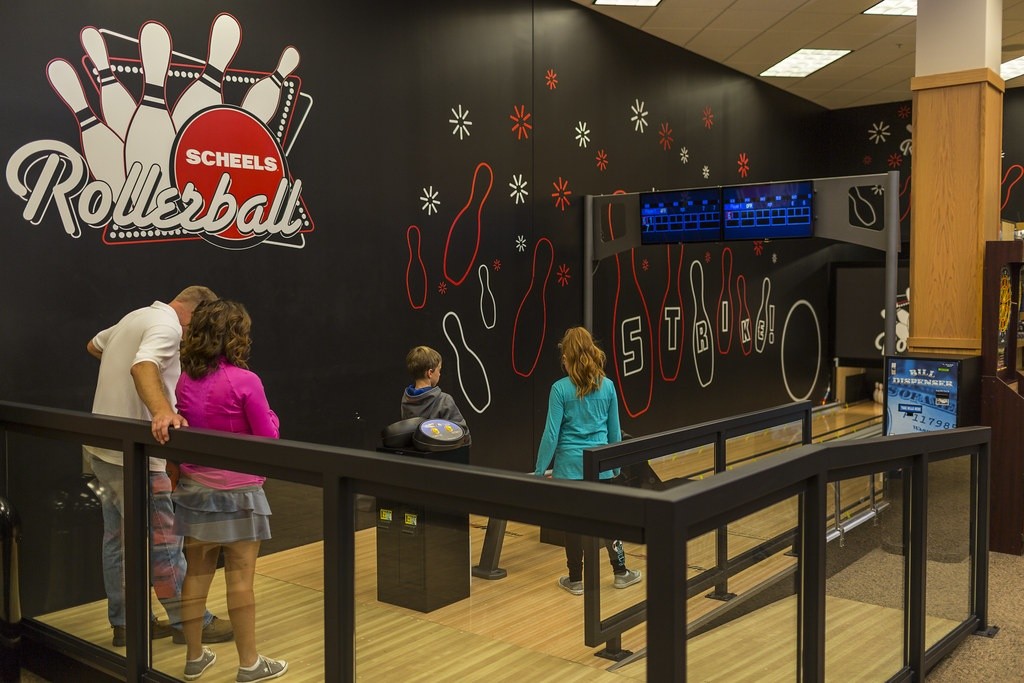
[172,616,233,644]
[614,568,641,588]
[183,647,217,680]
[235,654,288,683]
[111,615,172,646]
[559,575,584,594]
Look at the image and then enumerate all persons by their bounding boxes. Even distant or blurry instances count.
[174,298,290,683]
[401,346,471,443]
[535,328,642,595]
[83,287,233,647]
